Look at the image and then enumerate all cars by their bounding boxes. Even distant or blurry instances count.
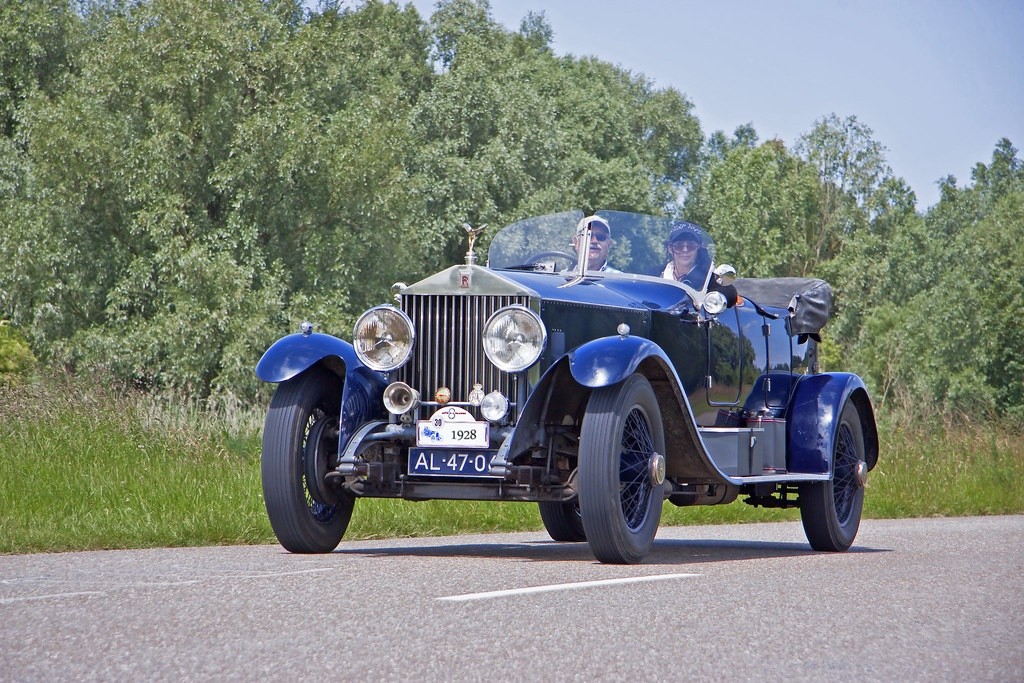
[253,207,883,564]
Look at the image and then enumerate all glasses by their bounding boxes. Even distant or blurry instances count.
[591,233,609,242]
[671,243,698,252]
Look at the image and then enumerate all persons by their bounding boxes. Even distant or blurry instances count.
[560,214,624,273]
[659,222,738,309]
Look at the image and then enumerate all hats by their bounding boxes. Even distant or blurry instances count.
[669,222,703,242]
[576,214,611,237]
